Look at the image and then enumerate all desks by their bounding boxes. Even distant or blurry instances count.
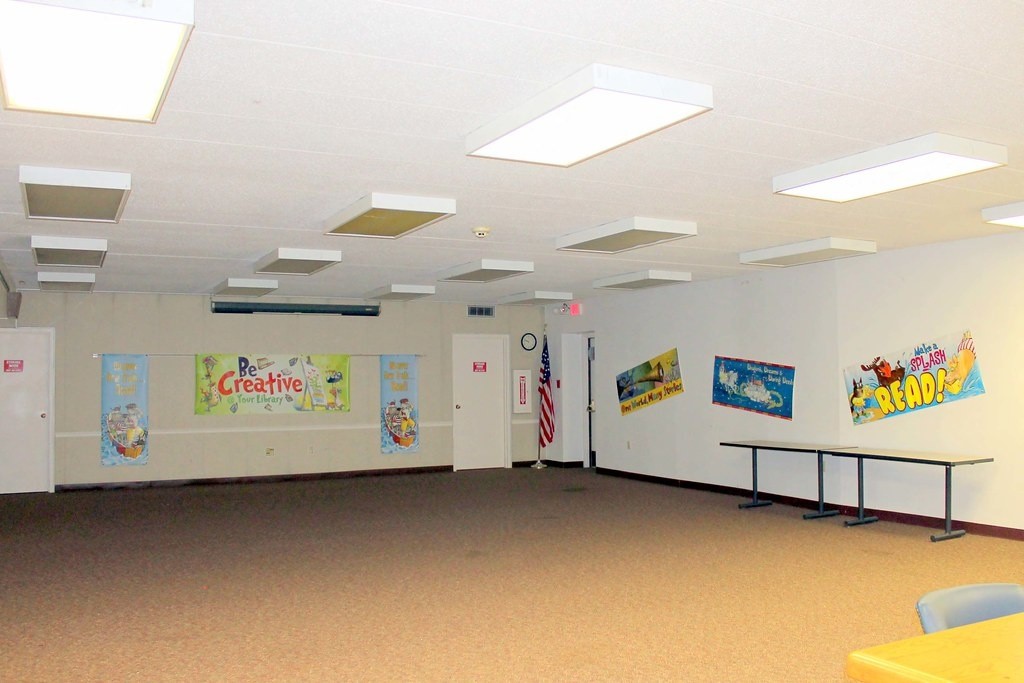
[719,441,858,521]
[845,611,1024,683]
[817,448,993,542]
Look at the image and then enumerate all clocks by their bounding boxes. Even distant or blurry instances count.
[521,333,537,352]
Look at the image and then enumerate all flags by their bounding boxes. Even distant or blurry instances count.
[537,335,556,448]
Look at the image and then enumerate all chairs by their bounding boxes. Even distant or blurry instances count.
[914,582,1024,632]
